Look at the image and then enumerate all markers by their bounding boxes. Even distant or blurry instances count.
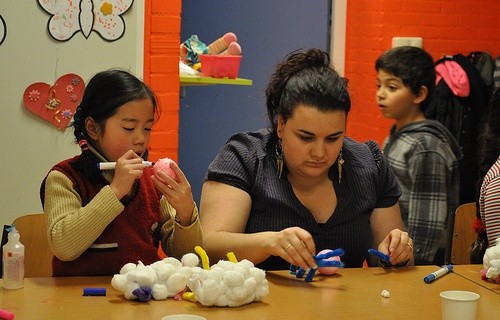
[97,160,155,170]
[424,264,454,283]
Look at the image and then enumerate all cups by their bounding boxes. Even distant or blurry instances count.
[161,314,206,320]
[439,290,480,320]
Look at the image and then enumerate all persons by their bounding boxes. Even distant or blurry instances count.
[375,45,464,265]
[199,48,414,271]
[470,85,500,265]
[40,67,203,276]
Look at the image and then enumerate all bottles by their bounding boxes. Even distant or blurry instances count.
[3,224,25,290]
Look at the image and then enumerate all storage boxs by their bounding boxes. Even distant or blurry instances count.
[200,53,242,76]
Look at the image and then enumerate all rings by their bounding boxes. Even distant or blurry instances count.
[285,244,292,251]
[408,243,412,251]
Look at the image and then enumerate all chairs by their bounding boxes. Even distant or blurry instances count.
[452,201,478,264]
[11,212,54,278]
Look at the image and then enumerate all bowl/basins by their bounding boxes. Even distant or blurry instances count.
[200,53,242,79]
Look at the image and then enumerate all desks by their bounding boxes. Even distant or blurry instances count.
[442,263,499,294]
[0,263,478,320]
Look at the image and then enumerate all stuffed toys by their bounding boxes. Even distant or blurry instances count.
[180,31,241,73]
[111,241,202,302]
[480,237,500,284]
[290,249,345,282]
[185,246,269,307]
